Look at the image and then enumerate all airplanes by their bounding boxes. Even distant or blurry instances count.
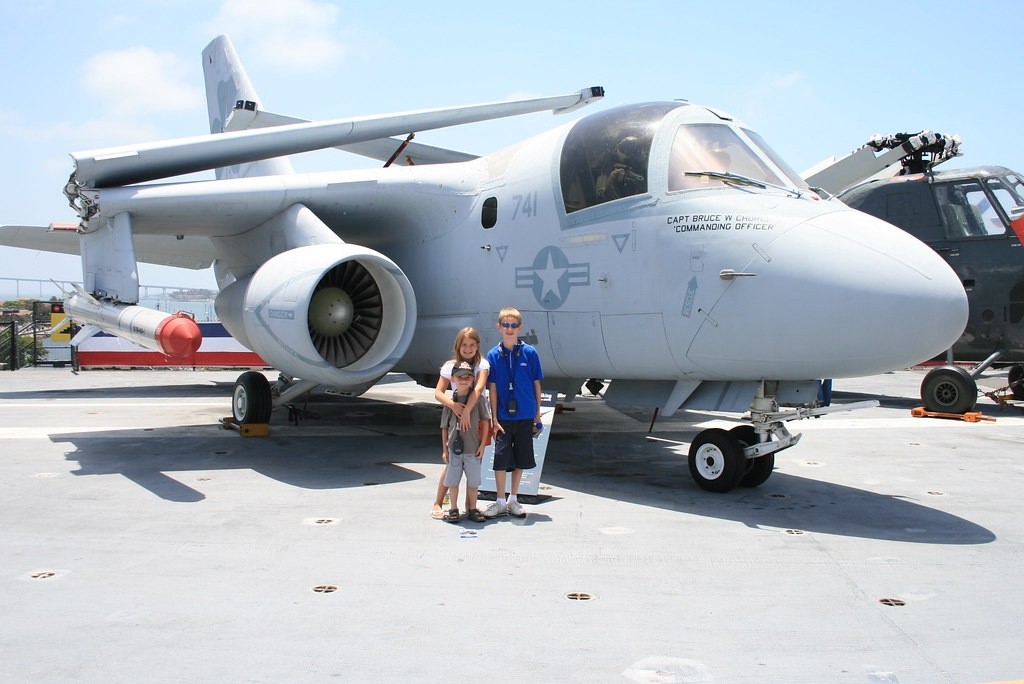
[0,30,971,493]
[840,164,1024,415]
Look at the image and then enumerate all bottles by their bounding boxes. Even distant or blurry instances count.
[532,422,542,437]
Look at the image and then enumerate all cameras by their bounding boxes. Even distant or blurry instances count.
[507,400,517,415]
[453,438,464,455]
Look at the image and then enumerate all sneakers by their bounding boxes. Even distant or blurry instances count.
[507,500,526,517]
[482,502,509,519]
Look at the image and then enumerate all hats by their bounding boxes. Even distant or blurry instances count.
[451,360,475,378]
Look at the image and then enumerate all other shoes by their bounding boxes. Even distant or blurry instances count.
[449,509,459,521]
[469,509,486,521]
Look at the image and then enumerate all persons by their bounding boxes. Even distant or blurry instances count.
[440,360,491,524]
[607,137,647,200]
[481,307,544,520]
[431,326,491,519]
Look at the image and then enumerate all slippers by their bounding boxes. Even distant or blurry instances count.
[432,504,444,519]
[462,511,469,518]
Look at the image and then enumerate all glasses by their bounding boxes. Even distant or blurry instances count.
[501,322,520,328]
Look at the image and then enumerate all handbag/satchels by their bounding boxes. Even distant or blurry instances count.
[478,419,493,446]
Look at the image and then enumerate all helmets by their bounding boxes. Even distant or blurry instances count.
[616,136,642,167]
[706,148,731,171]
